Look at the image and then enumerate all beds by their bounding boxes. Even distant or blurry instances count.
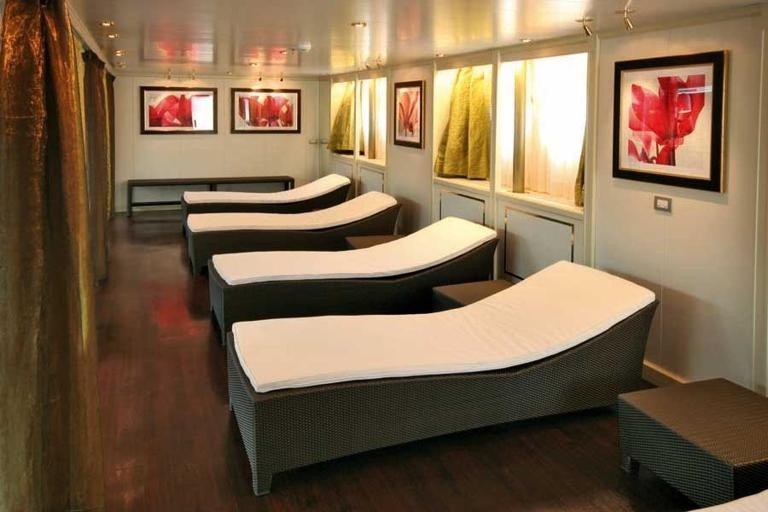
[686,489,768,512]
[230,262,658,495]
[209,215,502,347]
[187,189,404,280]
[184,174,352,226]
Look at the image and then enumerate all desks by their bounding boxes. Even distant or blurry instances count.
[432,276,514,311]
[343,236,401,249]
[617,377,768,506]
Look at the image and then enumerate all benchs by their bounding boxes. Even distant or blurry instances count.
[127,175,294,217]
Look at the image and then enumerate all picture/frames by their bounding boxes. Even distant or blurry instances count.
[140,86,218,134]
[231,87,301,134]
[393,80,424,149]
[612,50,727,193]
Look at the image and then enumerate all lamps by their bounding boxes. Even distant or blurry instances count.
[166,72,287,84]
[622,11,634,31]
[363,62,384,70]
[583,20,594,38]
